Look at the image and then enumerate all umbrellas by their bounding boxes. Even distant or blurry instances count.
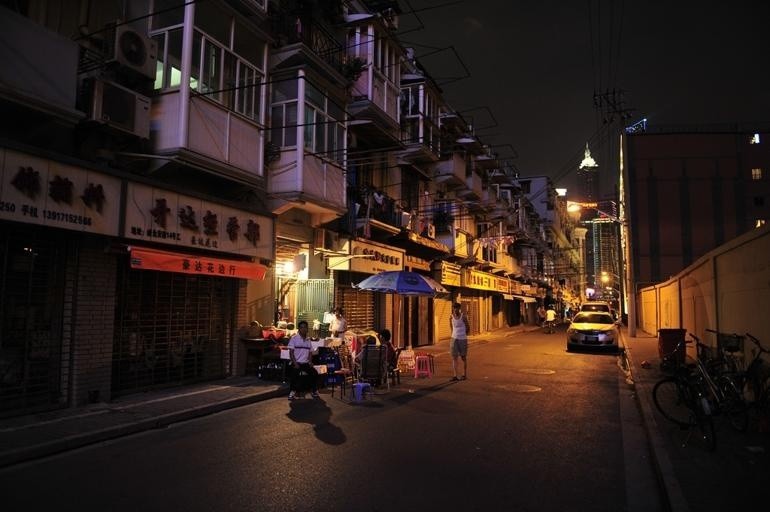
[354,271,448,349]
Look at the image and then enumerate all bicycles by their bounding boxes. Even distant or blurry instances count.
[653,323,768,450]
[547,321,555,335]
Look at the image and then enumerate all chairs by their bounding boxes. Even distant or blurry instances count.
[333,334,405,395]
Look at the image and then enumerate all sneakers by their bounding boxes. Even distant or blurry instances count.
[449,375,467,382]
[287,392,320,401]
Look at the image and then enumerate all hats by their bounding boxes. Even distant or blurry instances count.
[452,302,461,308]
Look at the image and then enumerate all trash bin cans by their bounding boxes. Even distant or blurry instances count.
[657,328,687,370]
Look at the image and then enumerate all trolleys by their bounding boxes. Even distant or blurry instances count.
[257,363,288,386]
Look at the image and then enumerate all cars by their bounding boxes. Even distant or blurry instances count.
[564,298,623,352]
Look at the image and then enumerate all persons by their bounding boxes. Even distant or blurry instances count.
[287,321,320,400]
[537,305,546,328]
[448,303,470,382]
[545,306,558,327]
[353,335,376,376]
[379,330,397,369]
[328,307,347,345]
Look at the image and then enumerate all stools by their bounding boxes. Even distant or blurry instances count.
[351,383,371,401]
[332,370,354,400]
[413,353,433,380]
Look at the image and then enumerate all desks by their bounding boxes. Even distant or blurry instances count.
[240,336,289,377]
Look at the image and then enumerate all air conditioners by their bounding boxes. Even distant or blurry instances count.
[77,77,152,141]
[313,227,339,257]
[101,18,159,81]
[396,208,412,232]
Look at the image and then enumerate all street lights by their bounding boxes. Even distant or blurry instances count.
[598,264,624,320]
[566,128,640,338]
